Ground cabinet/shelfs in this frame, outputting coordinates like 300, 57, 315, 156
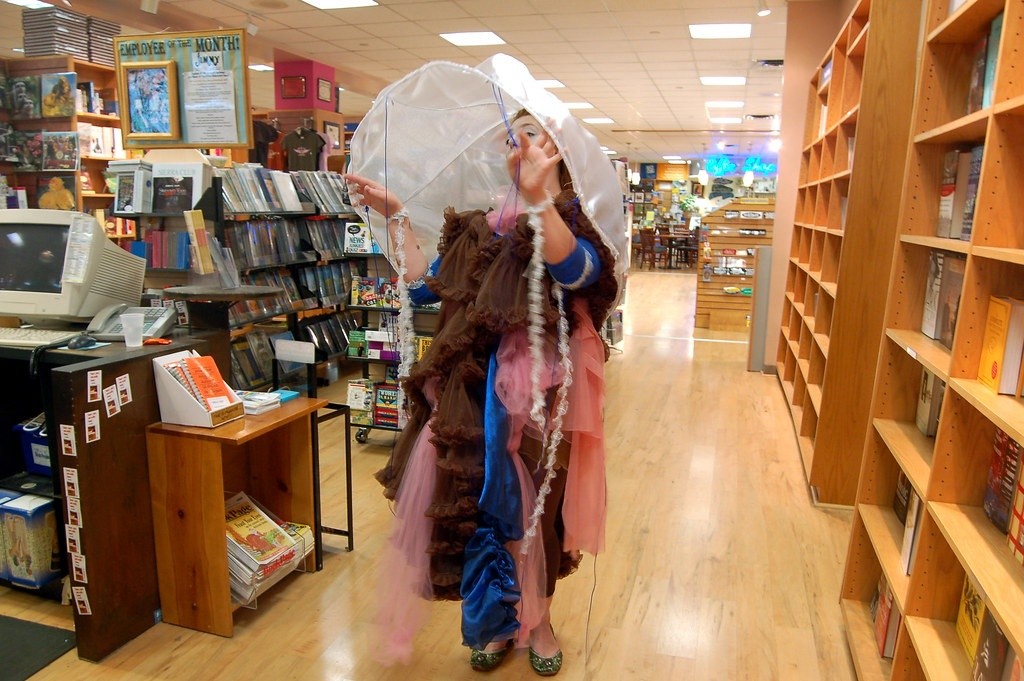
776, 0, 1024, 681
0, 55, 405, 443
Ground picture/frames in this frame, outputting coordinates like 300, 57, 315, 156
318, 77, 332, 102
323, 120, 341, 150
121, 59, 181, 141
281, 76, 305, 99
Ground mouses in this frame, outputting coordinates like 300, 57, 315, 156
68, 335, 97, 349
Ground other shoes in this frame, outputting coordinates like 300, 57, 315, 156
471, 636, 515, 671
529, 622, 563, 676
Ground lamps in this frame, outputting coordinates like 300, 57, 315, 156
244, 14, 258, 36
625, 142, 633, 181
698, 142, 709, 186
756, 0, 771, 17
742, 143, 754, 187
138, 0, 159, 15
632, 147, 640, 185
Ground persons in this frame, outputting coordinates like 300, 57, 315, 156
342, 107, 618, 676
3, 513, 33, 574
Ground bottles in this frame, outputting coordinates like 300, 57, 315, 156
643, 220, 647, 228
640, 218, 643, 229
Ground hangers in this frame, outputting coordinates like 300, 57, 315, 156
271, 119, 281, 134
294, 118, 314, 137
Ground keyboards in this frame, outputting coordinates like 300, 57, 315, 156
0, 327, 82, 347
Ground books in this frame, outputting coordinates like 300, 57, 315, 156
964, 10, 1004, 115
231, 388, 282, 415
956, 573, 1024, 681
981, 425, 1024, 566
867, 568, 902, 660
916, 365, 948, 440
162, 356, 235, 416
920, 250, 968, 351
892, 467, 923, 578
224, 489, 317, 601
936, 142, 985, 242
976, 292, 1024, 398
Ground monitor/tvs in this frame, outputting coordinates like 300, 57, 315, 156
0, 209, 147, 332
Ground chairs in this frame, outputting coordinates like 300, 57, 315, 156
639, 225, 698, 271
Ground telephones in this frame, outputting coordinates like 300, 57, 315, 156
86, 303, 178, 343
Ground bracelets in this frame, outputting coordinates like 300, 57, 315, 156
519, 186, 555, 439
387, 207, 417, 433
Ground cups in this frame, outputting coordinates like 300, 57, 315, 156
119, 313, 144, 347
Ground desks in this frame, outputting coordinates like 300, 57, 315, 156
664, 231, 691, 264
146, 390, 329, 636
650, 234, 694, 270
